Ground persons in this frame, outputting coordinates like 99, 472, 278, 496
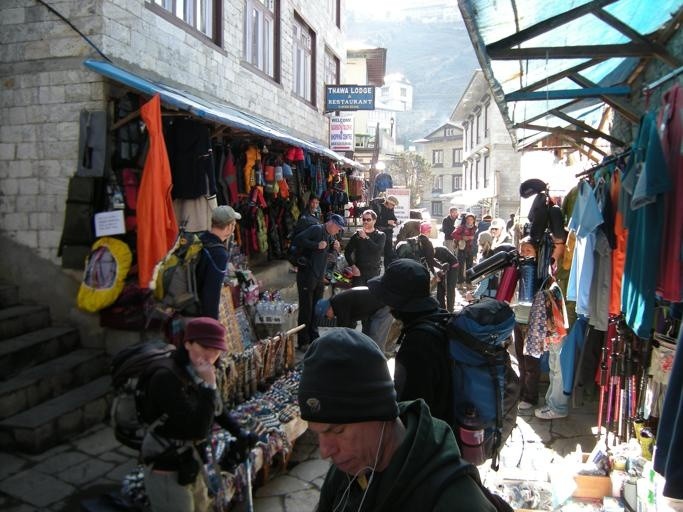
135, 317, 260, 511
293, 325, 515, 512
286, 196, 585, 474
162, 204, 242, 348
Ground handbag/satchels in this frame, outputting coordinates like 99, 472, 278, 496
100, 284, 165, 331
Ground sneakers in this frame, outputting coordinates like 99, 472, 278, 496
535, 406, 567, 419
519, 400, 532, 410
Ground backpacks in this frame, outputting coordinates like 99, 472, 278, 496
103, 338, 177, 449
144, 232, 223, 321
395, 237, 419, 258
453, 212, 477, 249
428, 299, 522, 471
287, 215, 326, 266
369, 198, 385, 210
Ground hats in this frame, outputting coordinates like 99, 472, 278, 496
332, 214, 346, 232
386, 196, 398, 205
212, 205, 242, 225
182, 316, 228, 351
490, 218, 502, 229
314, 299, 331, 317
520, 179, 566, 244
366, 258, 439, 312
420, 223, 431, 233
300, 328, 400, 423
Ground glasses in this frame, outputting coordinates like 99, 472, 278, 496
363, 218, 371, 222
